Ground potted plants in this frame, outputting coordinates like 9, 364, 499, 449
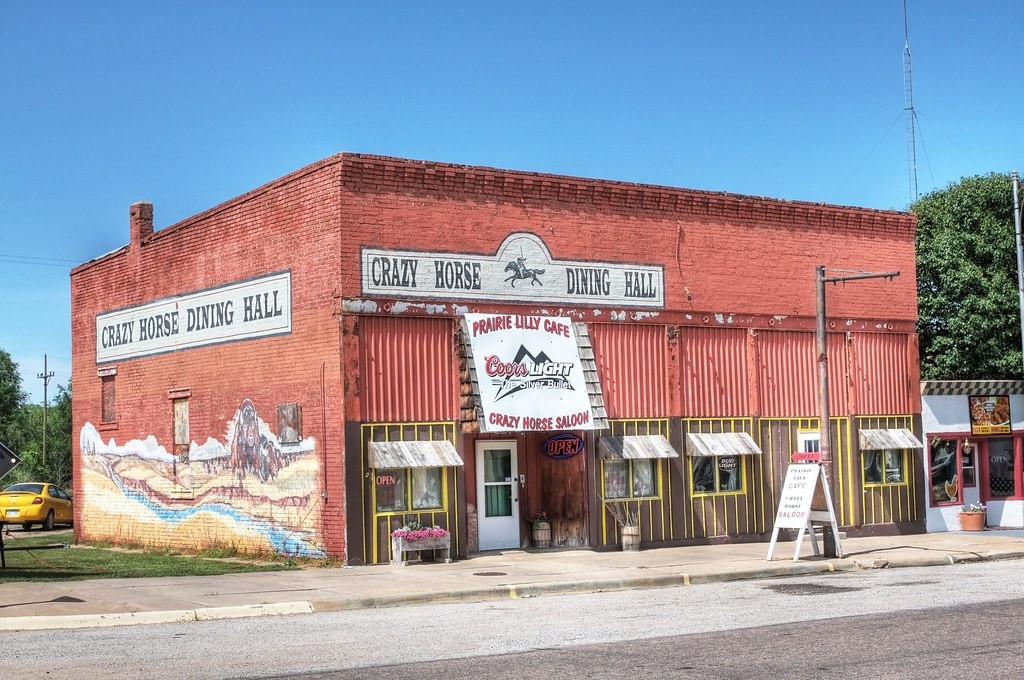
596, 489, 646, 553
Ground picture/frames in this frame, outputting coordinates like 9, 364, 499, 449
968, 394, 1013, 436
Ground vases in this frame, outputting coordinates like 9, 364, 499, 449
390, 533, 454, 567
960, 512, 986, 531
532, 522, 551, 548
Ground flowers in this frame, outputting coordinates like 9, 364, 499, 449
391, 522, 450, 542
526, 512, 551, 528
962, 501, 986, 512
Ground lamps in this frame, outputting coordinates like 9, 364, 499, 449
963, 437, 971, 455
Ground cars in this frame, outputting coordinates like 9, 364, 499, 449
0, 482, 73, 531
